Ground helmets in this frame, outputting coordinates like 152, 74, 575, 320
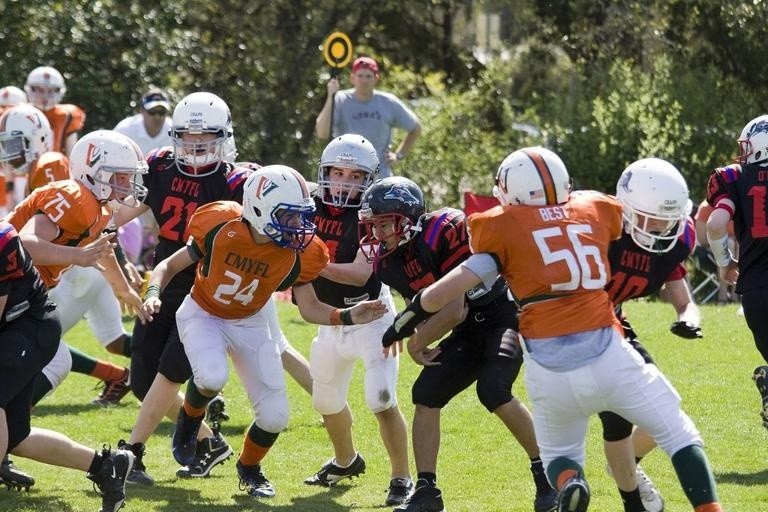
615, 158, 693, 254
355, 176, 425, 263
0, 65, 68, 177
69, 127, 149, 208
318, 132, 380, 210
736, 114, 768, 167
167, 92, 232, 178
493, 147, 569, 207
239, 162, 318, 251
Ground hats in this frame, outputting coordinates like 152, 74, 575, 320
352, 56, 379, 73
141, 89, 170, 111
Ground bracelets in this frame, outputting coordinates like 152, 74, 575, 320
142, 285, 162, 302
704, 230, 732, 267
394, 152, 405, 161
328, 306, 354, 327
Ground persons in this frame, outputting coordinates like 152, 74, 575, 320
312, 56, 424, 184
380, 144, 727, 512
0, 67, 265, 511
137, 164, 389, 499
290, 131, 417, 508
702, 113, 767, 438
597, 156, 706, 512
693, 198, 740, 307
355, 174, 561, 512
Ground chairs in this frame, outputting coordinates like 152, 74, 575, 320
685, 248, 733, 305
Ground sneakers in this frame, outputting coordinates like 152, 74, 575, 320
236, 458, 276, 497
532, 480, 591, 511
92, 365, 233, 512
750, 365, 767, 430
393, 486, 446, 512
306, 452, 365, 485
388, 475, 416, 504
608, 462, 665, 512
1, 458, 35, 492
